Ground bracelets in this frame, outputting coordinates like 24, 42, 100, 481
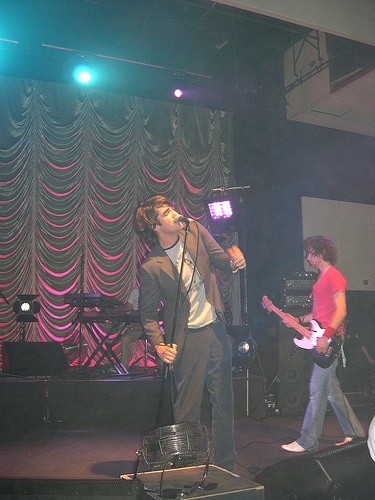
297, 317, 301, 324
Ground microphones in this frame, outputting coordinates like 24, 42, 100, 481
178, 215, 196, 223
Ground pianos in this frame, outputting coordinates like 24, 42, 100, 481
63, 293, 141, 376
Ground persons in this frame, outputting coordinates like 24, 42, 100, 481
109, 287, 167, 374
133, 196, 247, 472
281, 235, 367, 453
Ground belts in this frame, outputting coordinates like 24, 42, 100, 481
189, 319, 221, 332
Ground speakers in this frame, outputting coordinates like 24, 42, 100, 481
1, 339, 68, 377
276, 307, 312, 417
0, 476, 144, 500
252, 436, 375, 500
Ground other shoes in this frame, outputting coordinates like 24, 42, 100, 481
280, 440, 306, 452
334, 436, 354, 446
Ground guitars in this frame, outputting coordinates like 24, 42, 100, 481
260, 295, 342, 369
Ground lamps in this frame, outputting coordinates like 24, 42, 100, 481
13, 294, 41, 323
203, 194, 239, 235
232, 336, 257, 359
134, 422, 212, 496
